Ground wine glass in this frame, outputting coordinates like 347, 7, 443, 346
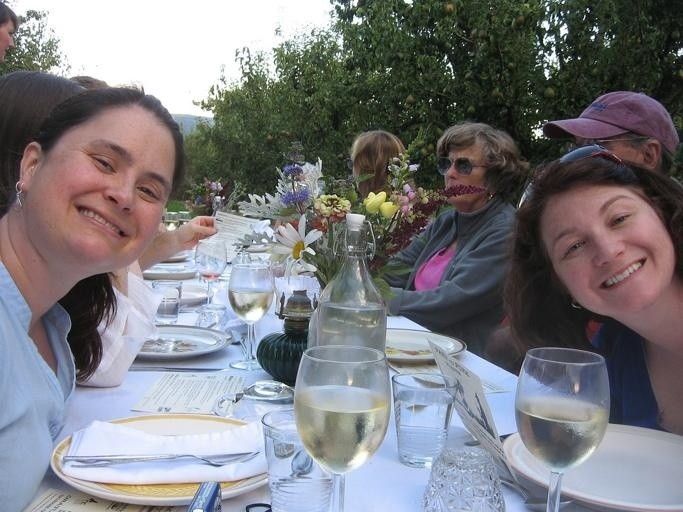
517, 348, 610, 512
296, 345, 392, 512
196, 241, 227, 304
229, 262, 275, 372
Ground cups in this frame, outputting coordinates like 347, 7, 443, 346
154, 279, 181, 326
199, 304, 229, 337
392, 372, 459, 464
426, 447, 506, 512
260, 409, 343, 510
214, 378, 296, 430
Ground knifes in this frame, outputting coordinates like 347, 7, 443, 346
130, 365, 224, 373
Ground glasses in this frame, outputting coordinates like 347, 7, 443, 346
434, 154, 491, 176
515, 143, 638, 211
564, 135, 659, 156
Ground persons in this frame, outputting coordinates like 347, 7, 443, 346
352, 130, 406, 196
69, 76, 219, 271
1, 69, 164, 388
0, 3, 19, 64
514, 146, 681, 431
0, 87, 185, 510
374, 119, 524, 355
486, 90, 681, 381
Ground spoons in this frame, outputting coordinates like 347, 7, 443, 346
61, 451, 255, 467
230, 328, 254, 361
498, 475, 577, 511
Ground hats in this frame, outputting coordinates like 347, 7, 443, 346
539, 87, 683, 157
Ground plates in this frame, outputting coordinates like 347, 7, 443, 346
385, 325, 465, 364
144, 251, 213, 305
137, 323, 230, 363
505, 422, 682, 510
48, 409, 271, 506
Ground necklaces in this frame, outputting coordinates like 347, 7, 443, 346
640, 340, 679, 428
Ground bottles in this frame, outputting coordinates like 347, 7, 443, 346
211, 196, 222, 216
305, 212, 385, 350
